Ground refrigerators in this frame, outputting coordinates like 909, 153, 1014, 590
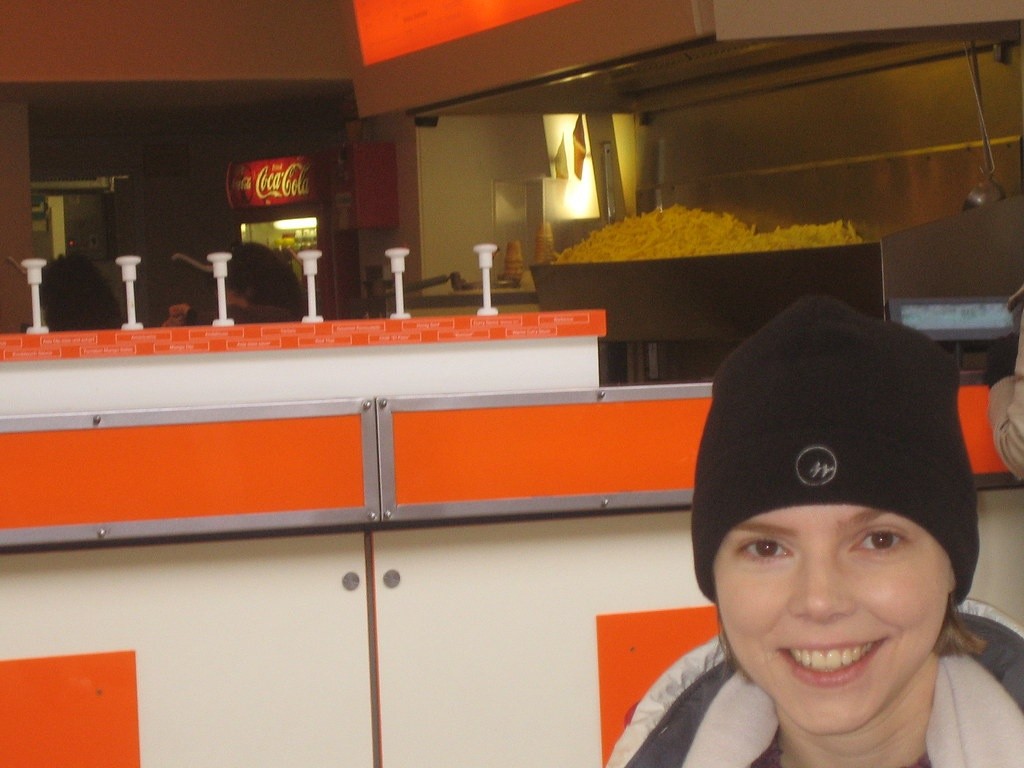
223, 153, 362, 320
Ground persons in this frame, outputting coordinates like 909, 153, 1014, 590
40, 242, 294, 333
605, 295, 1024, 768
985, 284, 1024, 481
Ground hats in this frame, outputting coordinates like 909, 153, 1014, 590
692, 299, 979, 605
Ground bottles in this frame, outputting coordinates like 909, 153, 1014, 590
270, 229, 317, 278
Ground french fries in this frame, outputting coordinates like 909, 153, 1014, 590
552, 204, 862, 263
